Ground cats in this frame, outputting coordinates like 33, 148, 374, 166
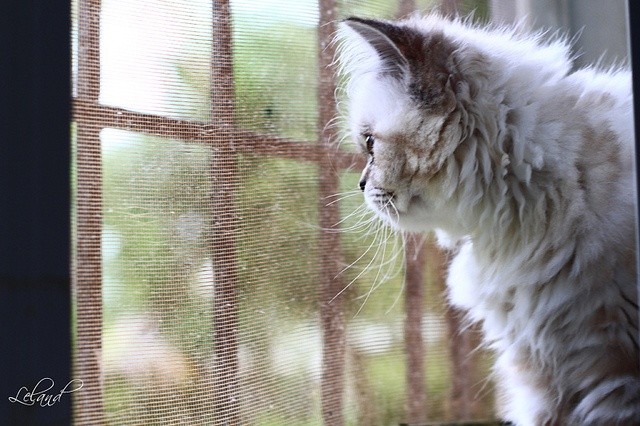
322, 4, 640, 426
